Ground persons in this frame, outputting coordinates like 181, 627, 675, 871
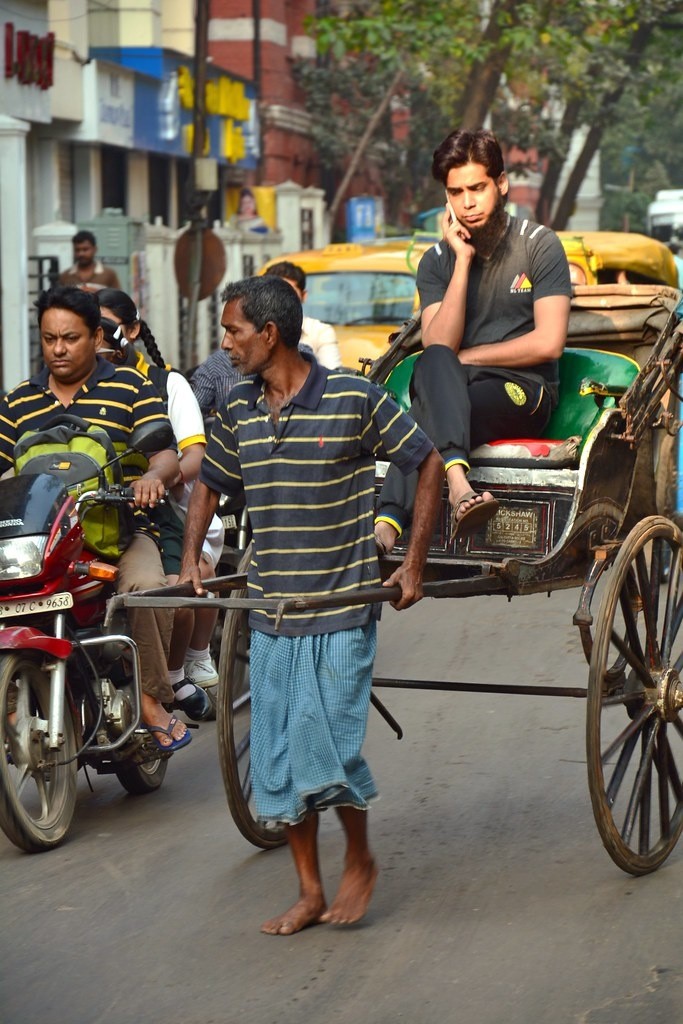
191, 341, 314, 414
58, 229, 122, 296
234, 189, 271, 234
173, 277, 445, 938
263, 262, 342, 371
0, 286, 225, 755
372, 126, 577, 557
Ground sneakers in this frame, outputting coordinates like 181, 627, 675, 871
186, 657, 220, 687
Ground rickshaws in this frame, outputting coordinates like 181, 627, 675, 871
105, 284, 682, 876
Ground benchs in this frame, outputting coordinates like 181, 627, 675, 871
381, 348, 642, 470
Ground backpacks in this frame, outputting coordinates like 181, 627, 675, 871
12, 414, 136, 557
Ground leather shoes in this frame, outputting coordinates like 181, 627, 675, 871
172, 676, 210, 719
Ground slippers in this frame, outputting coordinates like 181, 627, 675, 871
373, 533, 387, 558
140, 715, 193, 752
450, 491, 499, 539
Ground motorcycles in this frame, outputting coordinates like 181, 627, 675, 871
0, 422, 176, 852
253, 190, 683, 370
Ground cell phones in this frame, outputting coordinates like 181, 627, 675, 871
444, 190, 456, 223
